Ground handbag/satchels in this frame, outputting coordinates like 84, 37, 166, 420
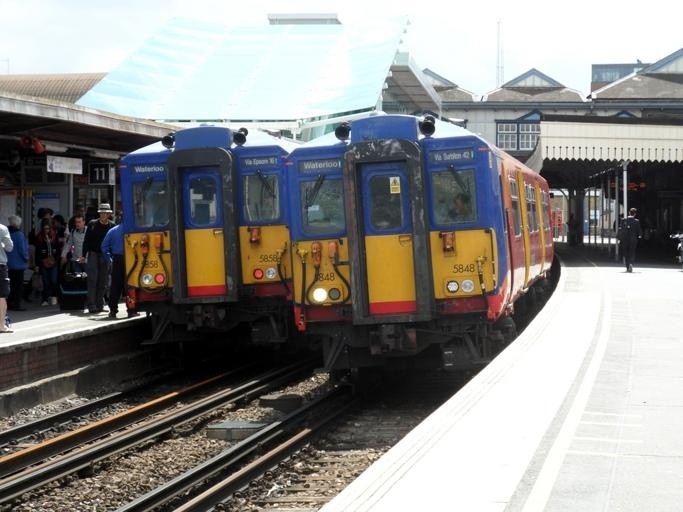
617, 220, 630, 240
43, 258, 53, 268
31, 274, 43, 287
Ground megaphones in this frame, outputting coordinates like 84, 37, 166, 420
32, 138, 45, 154
21, 135, 32, 148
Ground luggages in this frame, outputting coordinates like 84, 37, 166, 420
57, 260, 87, 311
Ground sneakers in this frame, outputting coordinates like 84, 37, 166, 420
51, 296, 57, 305
0, 327, 13, 333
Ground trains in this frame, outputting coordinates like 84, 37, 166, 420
285, 114, 555, 382
120, 125, 305, 368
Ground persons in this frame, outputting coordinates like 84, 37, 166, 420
5, 214, 30, 313
618, 207, 644, 273
447, 193, 476, 222
191, 185, 216, 225
31, 202, 142, 319
0, 223, 15, 334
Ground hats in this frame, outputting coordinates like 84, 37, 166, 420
97, 204, 112, 213
